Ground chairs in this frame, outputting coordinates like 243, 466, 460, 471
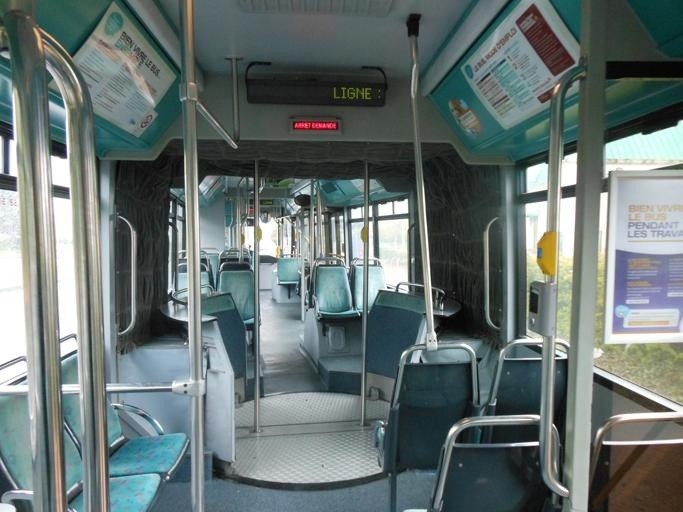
361, 283, 570, 512
588, 412, 683, 511
430, 415, 560, 512
1, 334, 192, 511
277, 253, 387, 334
173, 246, 255, 331
